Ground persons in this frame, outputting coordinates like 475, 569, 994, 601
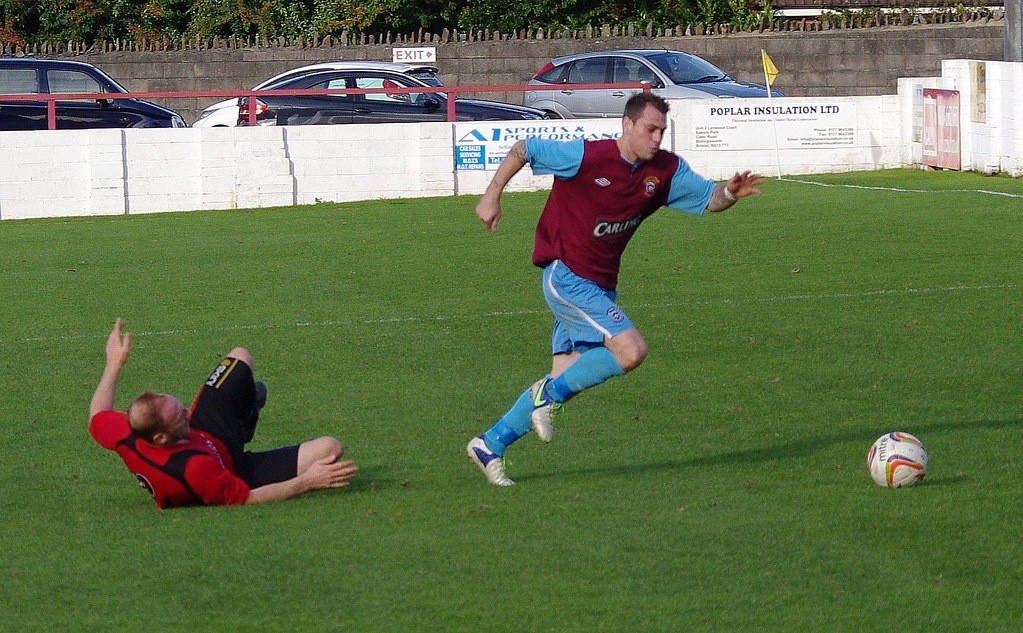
467, 93, 768, 484
87, 318, 357, 507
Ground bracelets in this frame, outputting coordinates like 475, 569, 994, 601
721, 186, 735, 201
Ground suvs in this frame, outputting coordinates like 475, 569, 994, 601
191, 60, 447, 129
233, 67, 551, 128
0, 54, 189, 131
522, 48, 786, 120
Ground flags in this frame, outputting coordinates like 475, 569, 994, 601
762, 50, 778, 86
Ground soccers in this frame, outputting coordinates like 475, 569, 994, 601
867, 431, 928, 488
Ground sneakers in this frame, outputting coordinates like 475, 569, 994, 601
466, 435, 514, 488
530, 375, 564, 443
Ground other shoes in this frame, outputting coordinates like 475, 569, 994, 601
254, 381, 267, 408
